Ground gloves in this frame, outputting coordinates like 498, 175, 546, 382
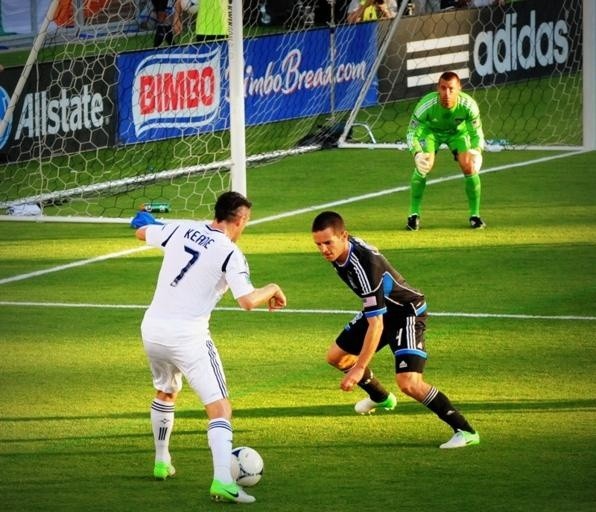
468, 149, 482, 172
415, 153, 430, 172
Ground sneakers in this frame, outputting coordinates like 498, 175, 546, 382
439, 431, 480, 449
408, 214, 419, 231
209, 480, 256, 504
469, 216, 485, 229
354, 392, 397, 415
154, 462, 175, 480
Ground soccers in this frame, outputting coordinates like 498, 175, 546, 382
227, 446, 263, 485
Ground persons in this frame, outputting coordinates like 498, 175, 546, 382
135, 192, 287, 503
311, 211, 480, 449
407, 71, 486, 230
244, 1, 398, 32
52, 0, 233, 47
